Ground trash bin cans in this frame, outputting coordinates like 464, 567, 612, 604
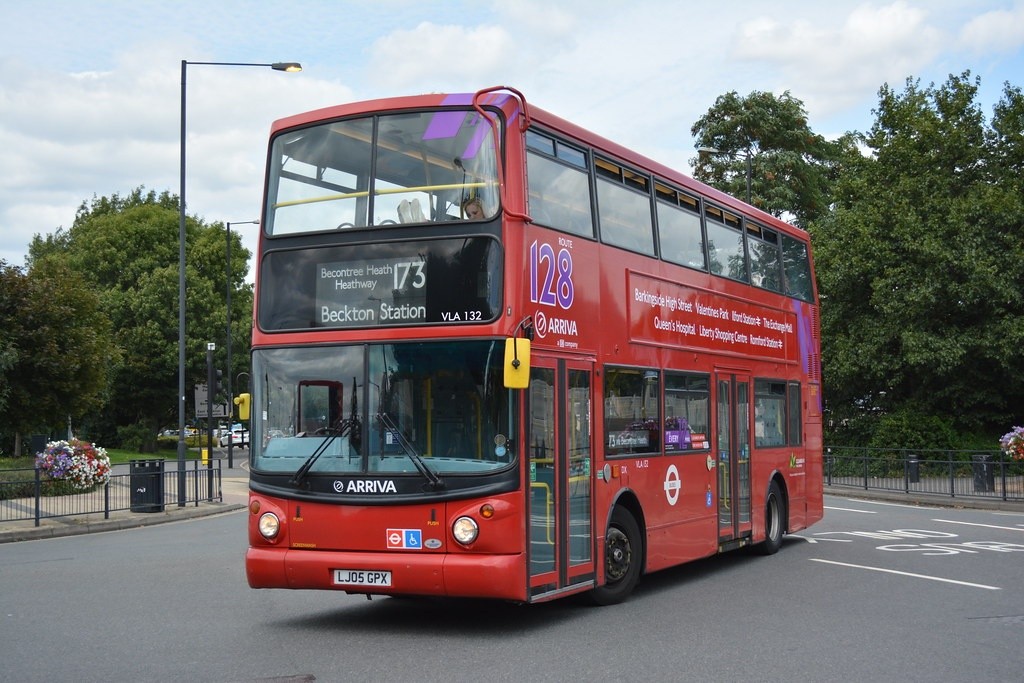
909, 454, 919, 484
129, 459, 165, 513
972, 454, 995, 492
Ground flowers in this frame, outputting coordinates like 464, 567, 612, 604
998, 427, 1024, 460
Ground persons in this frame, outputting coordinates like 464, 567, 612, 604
464, 197, 494, 221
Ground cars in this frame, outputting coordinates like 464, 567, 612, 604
158, 419, 251, 449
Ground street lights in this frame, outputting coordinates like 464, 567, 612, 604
177, 58, 302, 508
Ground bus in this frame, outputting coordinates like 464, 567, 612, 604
232, 84, 824, 607
